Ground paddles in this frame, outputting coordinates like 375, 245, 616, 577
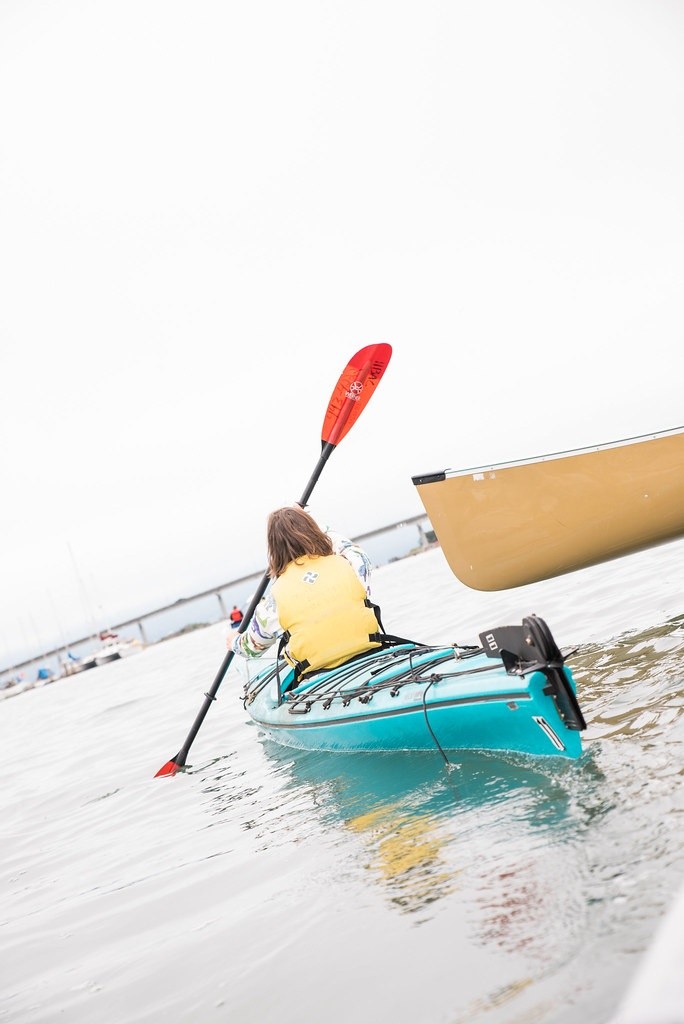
152, 342, 397, 780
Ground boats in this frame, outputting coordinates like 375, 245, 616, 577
237, 617, 589, 764
410, 423, 682, 593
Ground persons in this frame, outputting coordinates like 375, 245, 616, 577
230, 605, 243, 623
226, 506, 385, 693
